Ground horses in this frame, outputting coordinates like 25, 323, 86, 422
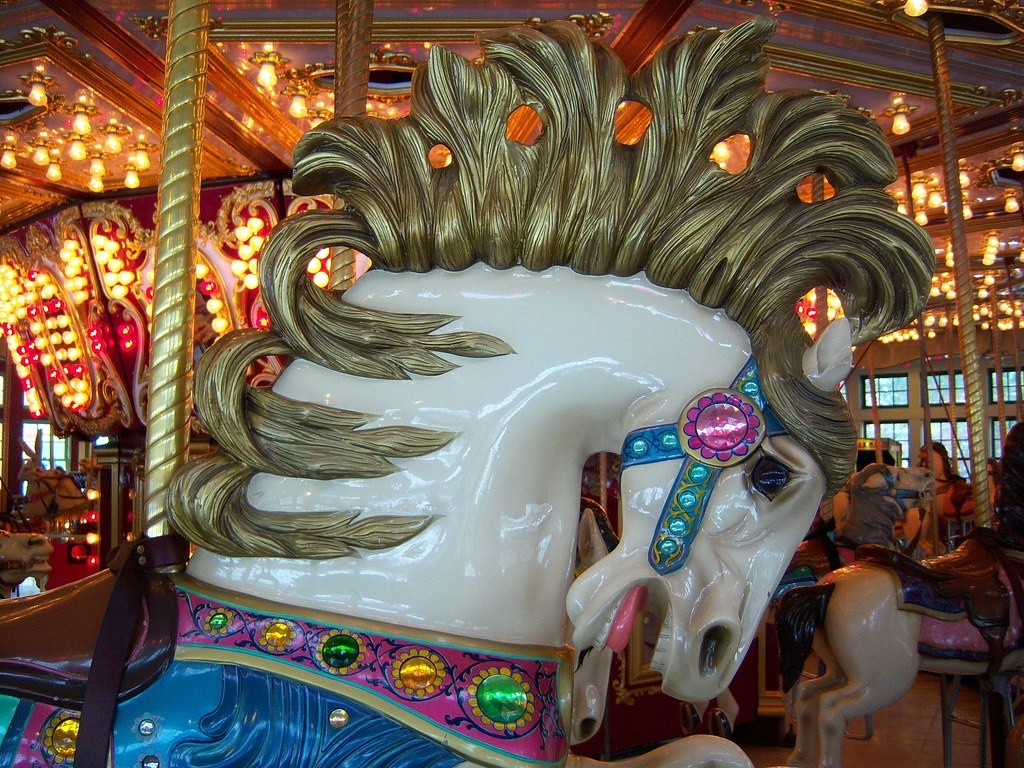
0, 15, 1024, 768
